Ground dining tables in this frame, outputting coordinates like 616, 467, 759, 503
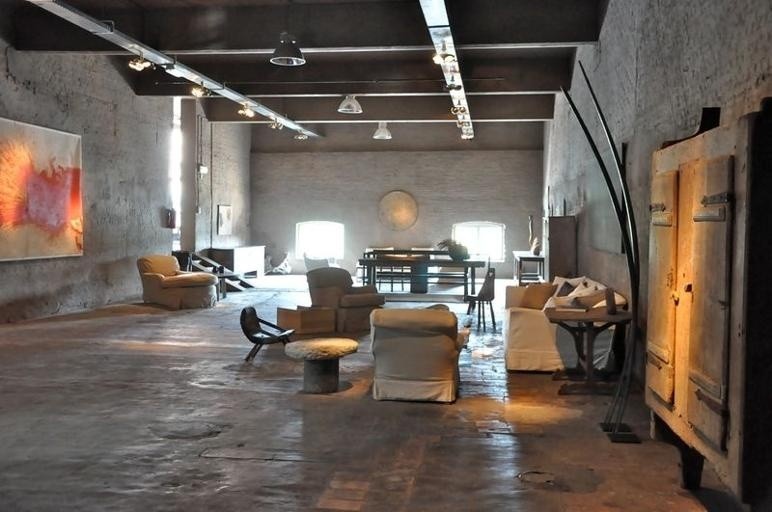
438, 239, 467, 261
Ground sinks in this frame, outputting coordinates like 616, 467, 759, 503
371, 308, 467, 405
306, 267, 384, 338
502, 277, 626, 373
136, 253, 219, 310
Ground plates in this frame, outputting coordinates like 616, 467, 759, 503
544, 216, 577, 282
644, 95, 772, 511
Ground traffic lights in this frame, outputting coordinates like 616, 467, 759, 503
543, 282, 586, 310
574, 289, 606, 307
520, 282, 557, 309
556, 281, 574, 297
553, 285, 596, 309
551, 276, 586, 296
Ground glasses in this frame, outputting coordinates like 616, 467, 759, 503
237, 102, 255, 118
442, 76, 462, 93
129, 54, 151, 71
269, 31, 306, 66
338, 97, 363, 114
432, 44, 455, 65
192, 82, 204, 97
267, 120, 283, 130
451, 100, 466, 115
373, 123, 392, 140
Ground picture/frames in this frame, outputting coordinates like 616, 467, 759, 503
545, 308, 631, 394
208, 246, 266, 278
513, 249, 544, 285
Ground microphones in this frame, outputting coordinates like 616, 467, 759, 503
285, 336, 357, 393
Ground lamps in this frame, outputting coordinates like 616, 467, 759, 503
464, 267, 495, 328
240, 307, 296, 361
